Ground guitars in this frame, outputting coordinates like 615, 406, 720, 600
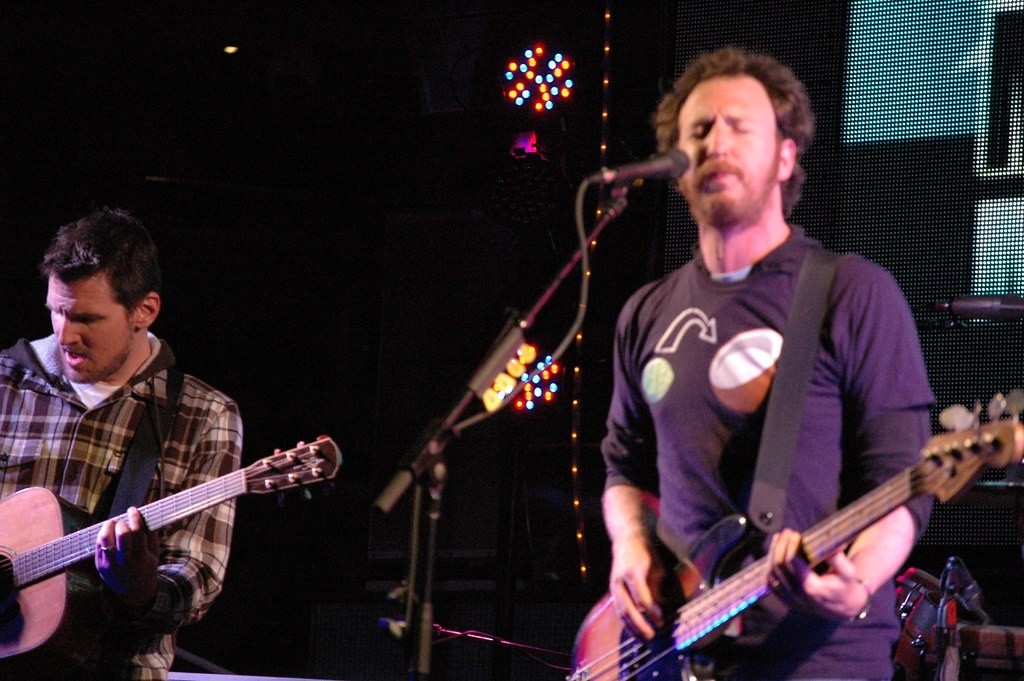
1, 434, 341, 662
566, 389, 1024, 681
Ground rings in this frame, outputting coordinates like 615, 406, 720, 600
98, 543, 116, 554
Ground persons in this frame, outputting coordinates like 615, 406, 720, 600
0, 212, 243, 681
601, 48, 933, 681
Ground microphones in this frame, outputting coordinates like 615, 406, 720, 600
589, 149, 689, 183
950, 558, 985, 611
937, 295, 1024, 322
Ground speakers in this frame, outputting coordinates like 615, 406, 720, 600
312, 208, 611, 681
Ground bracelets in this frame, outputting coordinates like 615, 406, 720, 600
846, 580, 874, 627
